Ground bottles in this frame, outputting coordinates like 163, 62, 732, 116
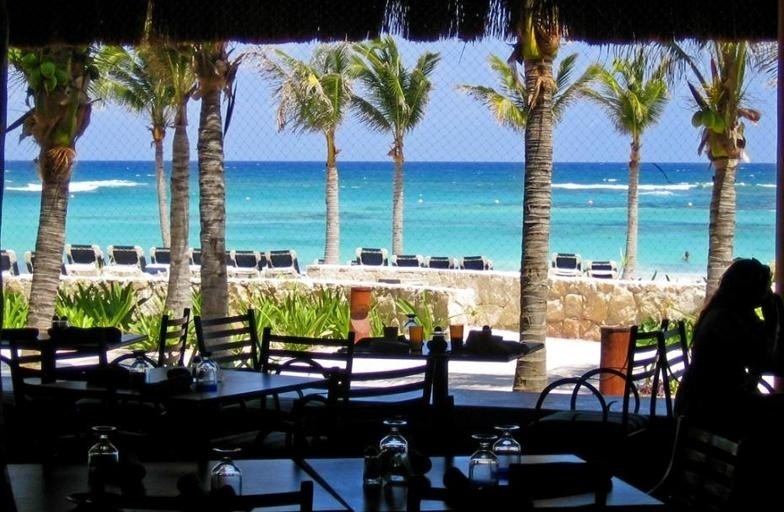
46, 316, 68, 336
128, 347, 222, 395
427, 326, 447, 351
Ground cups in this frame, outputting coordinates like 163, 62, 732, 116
448, 324, 463, 347
382, 326, 398, 341
408, 325, 424, 352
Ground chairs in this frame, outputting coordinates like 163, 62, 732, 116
0, 316, 436, 458
0, 243, 617, 281
530, 316, 783, 511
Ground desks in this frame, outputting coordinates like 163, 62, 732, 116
6, 458, 350, 511
303, 454, 665, 511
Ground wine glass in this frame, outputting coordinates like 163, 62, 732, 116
208, 447, 244, 496
85, 424, 120, 488
375, 417, 407, 463
490, 424, 524, 466
467, 431, 500, 482
403, 313, 418, 340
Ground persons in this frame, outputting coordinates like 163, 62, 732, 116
675, 259, 780, 499
